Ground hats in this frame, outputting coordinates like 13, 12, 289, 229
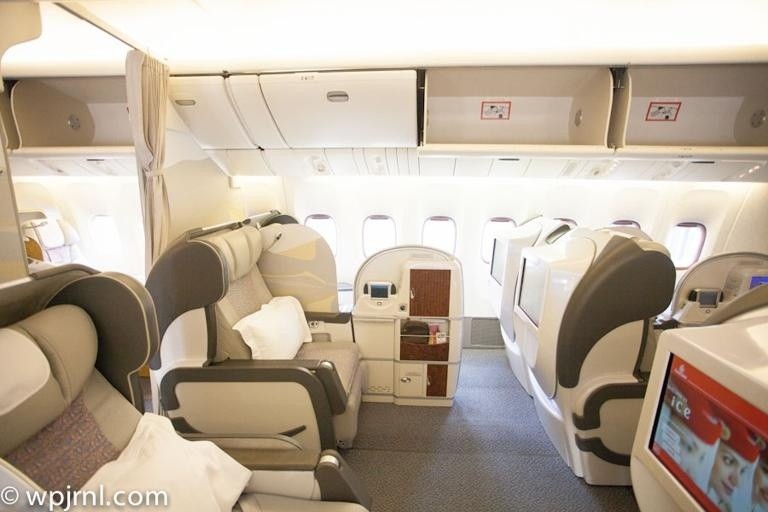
665, 382, 760, 462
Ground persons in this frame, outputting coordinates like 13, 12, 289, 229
668, 409, 768, 512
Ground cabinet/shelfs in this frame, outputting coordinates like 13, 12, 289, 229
349, 246, 465, 407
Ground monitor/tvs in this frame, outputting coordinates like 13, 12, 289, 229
514, 249, 551, 336
634, 331, 768, 512
488, 236, 509, 289
